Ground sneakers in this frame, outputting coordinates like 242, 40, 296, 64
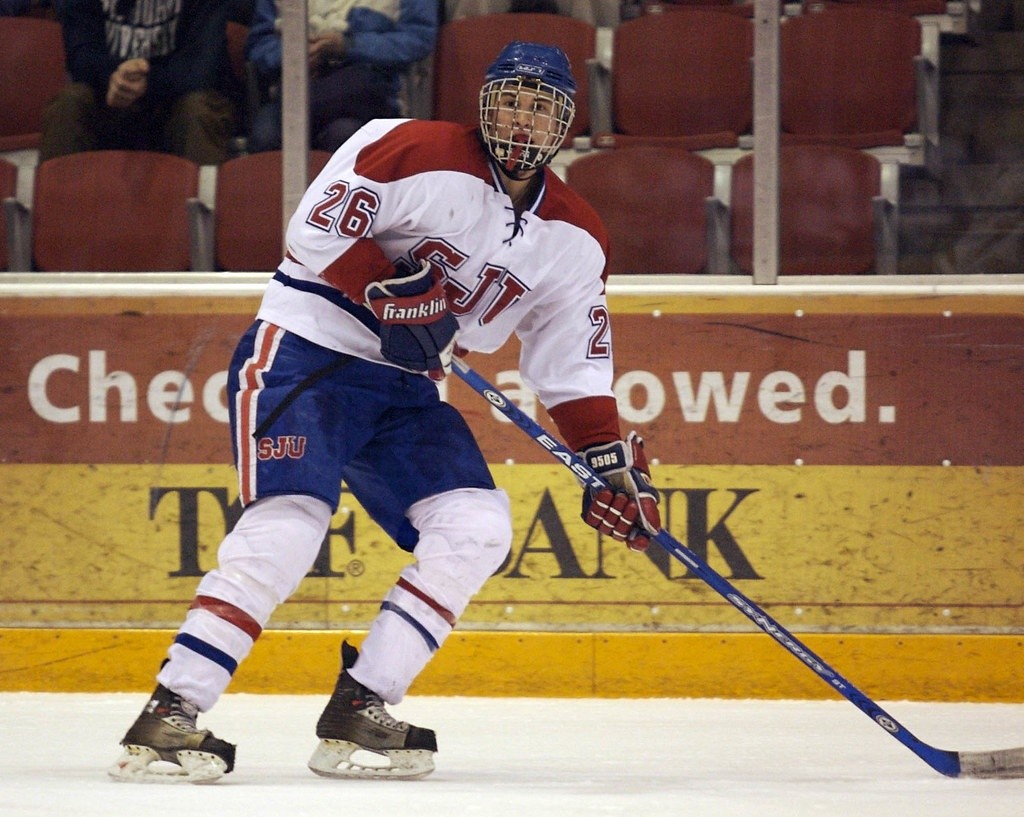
307, 639, 439, 781
106, 657, 237, 784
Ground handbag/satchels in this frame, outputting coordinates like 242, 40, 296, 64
307, 52, 402, 120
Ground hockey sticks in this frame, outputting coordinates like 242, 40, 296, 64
448, 352, 1024, 784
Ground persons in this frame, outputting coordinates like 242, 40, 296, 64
109, 39, 663, 773
41, 1, 441, 168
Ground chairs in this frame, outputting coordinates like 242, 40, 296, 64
32, 150, 217, 273
595, 13, 755, 151
728, 143, 898, 275
0, 16, 80, 153
0, 159, 34, 272
565, 145, 730, 273
780, 11, 943, 179
215, 150, 330, 272
433, 15, 612, 149
802, 0, 984, 43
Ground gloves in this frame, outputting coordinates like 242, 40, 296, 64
574, 430, 661, 553
362, 258, 461, 383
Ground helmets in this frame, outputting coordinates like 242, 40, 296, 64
478, 40, 577, 181
